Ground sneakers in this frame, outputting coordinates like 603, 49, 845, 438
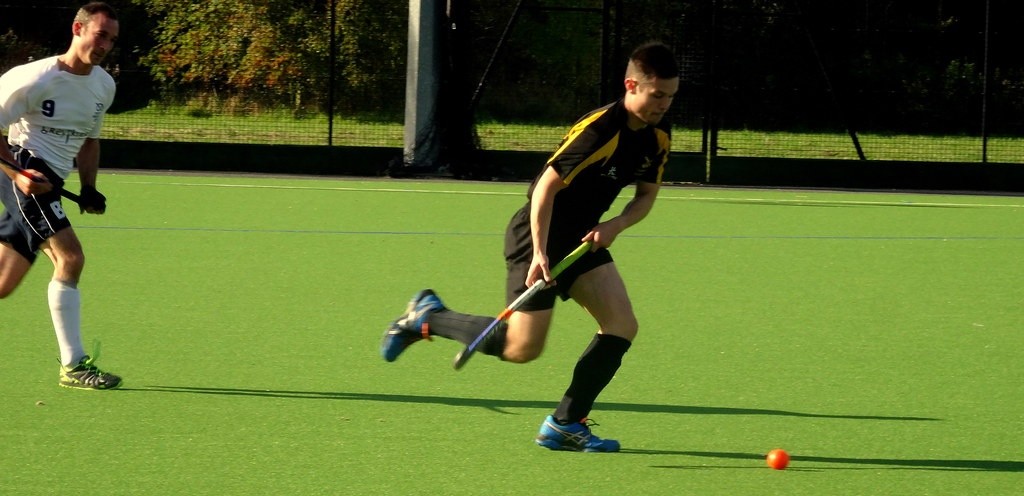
56, 339, 124, 392
380, 289, 445, 362
534, 415, 620, 454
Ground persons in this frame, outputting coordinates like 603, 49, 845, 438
0, 3, 123, 390
384, 43, 681, 453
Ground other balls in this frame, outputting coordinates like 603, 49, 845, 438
766, 448, 789, 470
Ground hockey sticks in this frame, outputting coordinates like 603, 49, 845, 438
451, 238, 594, 371
0, 156, 85, 206
